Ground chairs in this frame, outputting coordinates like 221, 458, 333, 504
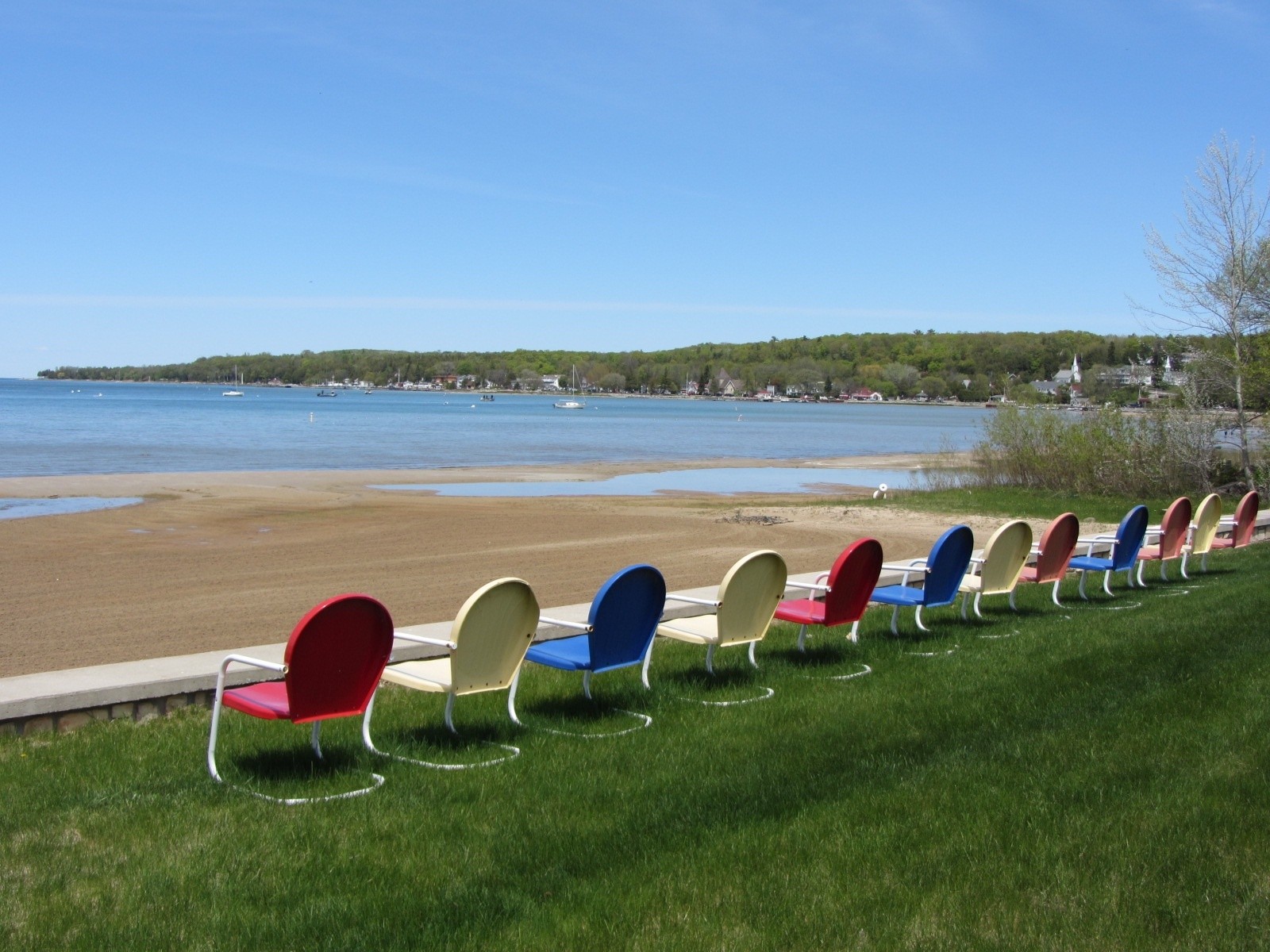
853, 524, 976, 659
1077, 503, 1151, 613
363, 578, 538, 769
1210, 491, 1260, 583
1135, 498, 1191, 602
959, 512, 1085, 627
747, 538, 883, 683
1177, 495, 1222, 589
507, 566, 665, 742
203, 593, 396, 805
643, 549, 788, 708
914, 520, 1033, 639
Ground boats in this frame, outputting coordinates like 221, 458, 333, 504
479, 393, 495, 402
364, 390, 373, 395
317, 390, 337, 397
985, 395, 1008, 408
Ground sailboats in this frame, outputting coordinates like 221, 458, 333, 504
222, 364, 244, 397
552, 363, 587, 409
